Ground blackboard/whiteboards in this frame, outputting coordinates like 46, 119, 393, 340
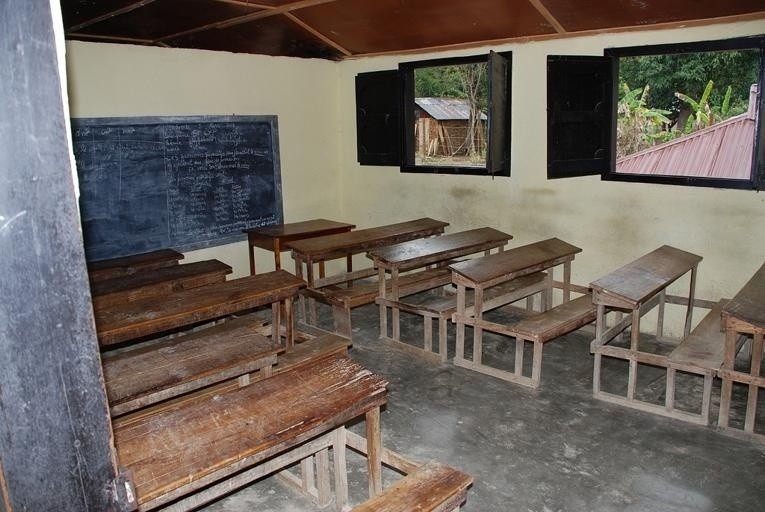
71, 115, 283, 264
